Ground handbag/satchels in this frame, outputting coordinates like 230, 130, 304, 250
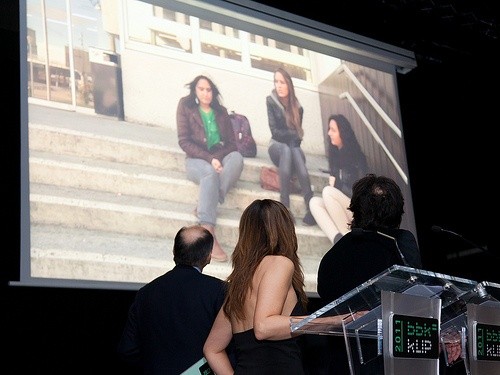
260, 167, 298, 193
229, 111, 256, 158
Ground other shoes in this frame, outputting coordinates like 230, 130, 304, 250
200, 224, 228, 262
302, 211, 316, 226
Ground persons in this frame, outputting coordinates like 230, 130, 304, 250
318, 177, 421, 308
120, 228, 235, 375
175, 75, 244, 263
309, 114, 366, 246
268, 70, 319, 228
203, 200, 372, 375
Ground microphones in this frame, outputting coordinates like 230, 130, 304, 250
352, 228, 409, 267
431, 225, 486, 252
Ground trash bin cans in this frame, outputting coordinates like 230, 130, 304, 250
89, 59, 119, 116
